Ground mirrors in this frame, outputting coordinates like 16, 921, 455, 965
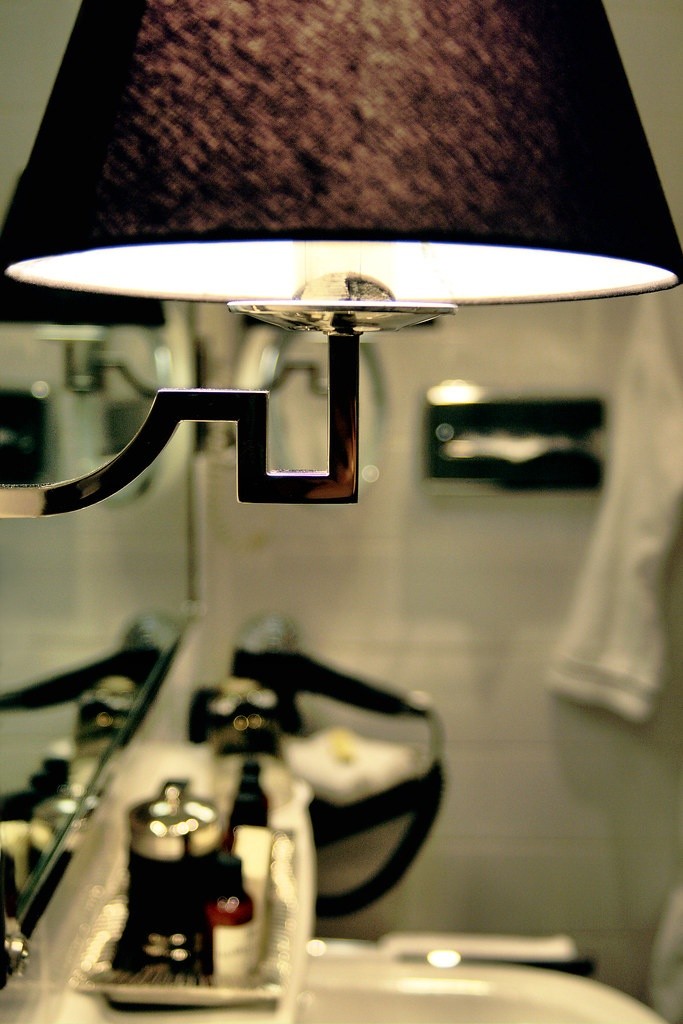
0, 0, 199, 986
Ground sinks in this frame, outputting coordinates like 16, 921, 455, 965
294, 942, 670, 1022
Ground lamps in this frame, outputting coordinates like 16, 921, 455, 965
0, 0, 683, 520
